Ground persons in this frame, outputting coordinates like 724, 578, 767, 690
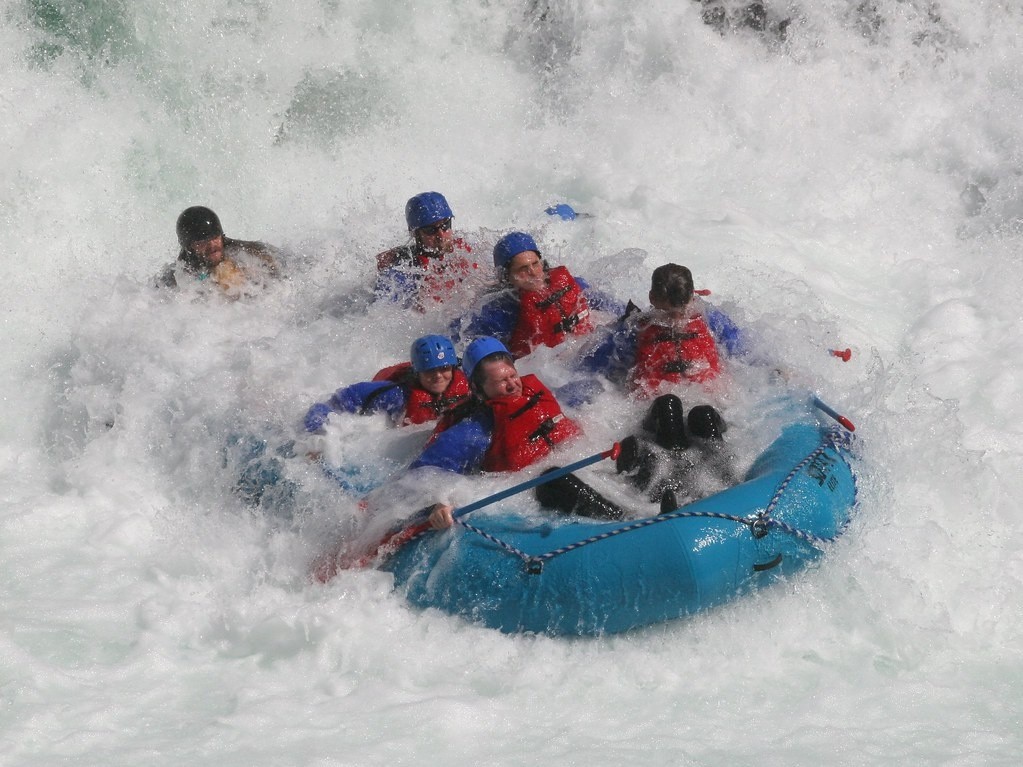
306, 335, 470, 426
377, 190, 470, 315
154, 204, 279, 288
577, 263, 742, 451
450, 232, 619, 359
409, 337, 676, 531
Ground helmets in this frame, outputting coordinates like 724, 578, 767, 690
493, 232, 541, 267
462, 337, 511, 381
176, 206, 223, 241
405, 191, 455, 231
545, 204, 576, 221
410, 334, 459, 373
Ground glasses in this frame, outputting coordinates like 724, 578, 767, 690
418, 219, 450, 236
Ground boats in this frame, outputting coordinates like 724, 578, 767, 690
222, 330, 888, 638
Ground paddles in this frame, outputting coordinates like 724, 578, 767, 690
813, 396, 855, 432
317, 443, 622, 581
829, 348, 851, 362
694, 289, 711, 295
